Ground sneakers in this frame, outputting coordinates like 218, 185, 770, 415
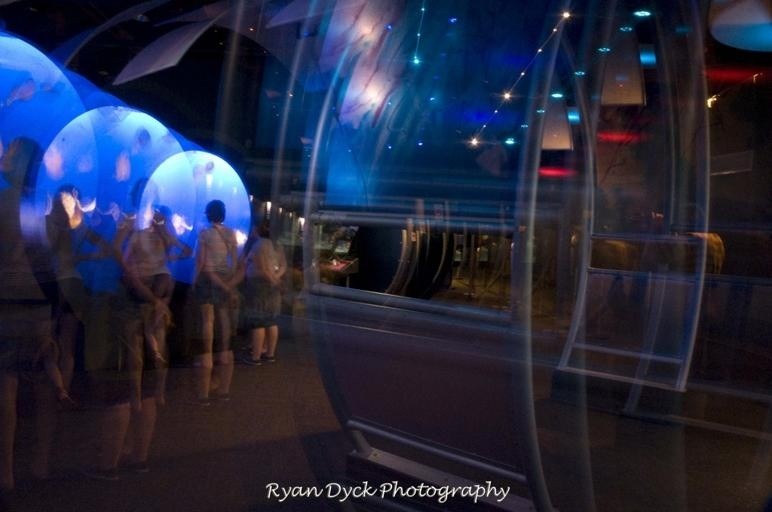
184, 345, 276, 407
82, 459, 150, 482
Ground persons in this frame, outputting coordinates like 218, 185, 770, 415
2, 134, 771, 485
